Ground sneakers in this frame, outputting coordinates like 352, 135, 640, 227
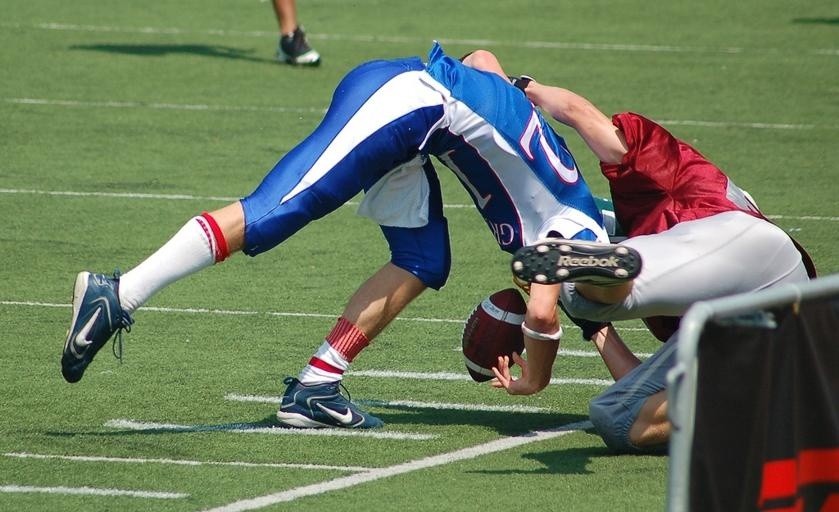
512, 236, 642, 288
277, 26, 320, 65
62, 267, 134, 383
277, 375, 385, 429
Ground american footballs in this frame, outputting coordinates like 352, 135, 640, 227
461, 289, 527, 382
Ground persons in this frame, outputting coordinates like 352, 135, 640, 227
274, 0, 320, 65
61, 38, 612, 432
504, 68, 818, 455
